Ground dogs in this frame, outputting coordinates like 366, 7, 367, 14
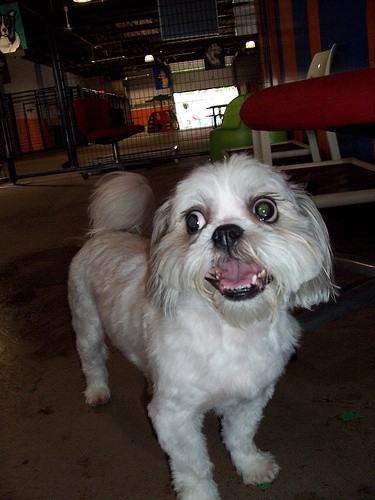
66, 149, 341, 500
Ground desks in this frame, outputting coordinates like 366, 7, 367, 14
207, 104, 229, 129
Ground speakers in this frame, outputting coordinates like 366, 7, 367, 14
157, 0, 219, 42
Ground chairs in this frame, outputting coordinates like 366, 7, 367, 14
239, 0, 375, 208
250, 42, 342, 169
209, 93, 288, 162
73, 97, 159, 179
